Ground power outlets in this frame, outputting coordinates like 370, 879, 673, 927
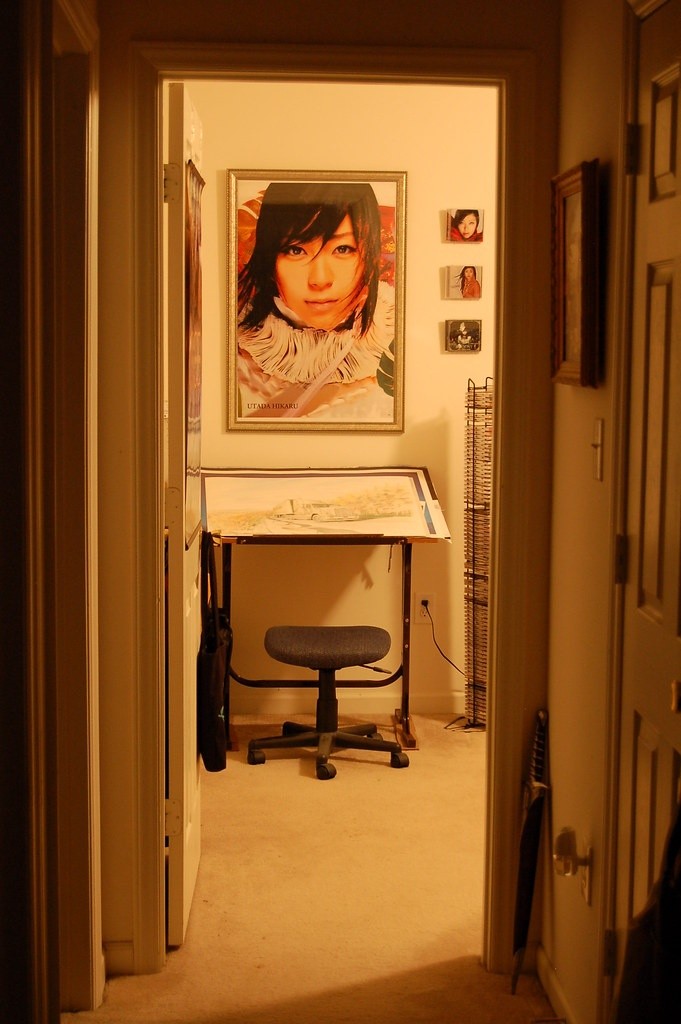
415, 593, 434, 624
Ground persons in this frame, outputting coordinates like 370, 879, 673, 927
453, 266, 480, 299
451, 209, 483, 242
458, 322, 477, 351
238, 184, 395, 422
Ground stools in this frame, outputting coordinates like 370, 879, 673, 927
250, 626, 410, 779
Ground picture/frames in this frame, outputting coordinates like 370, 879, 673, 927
226, 168, 406, 431
548, 159, 607, 390
